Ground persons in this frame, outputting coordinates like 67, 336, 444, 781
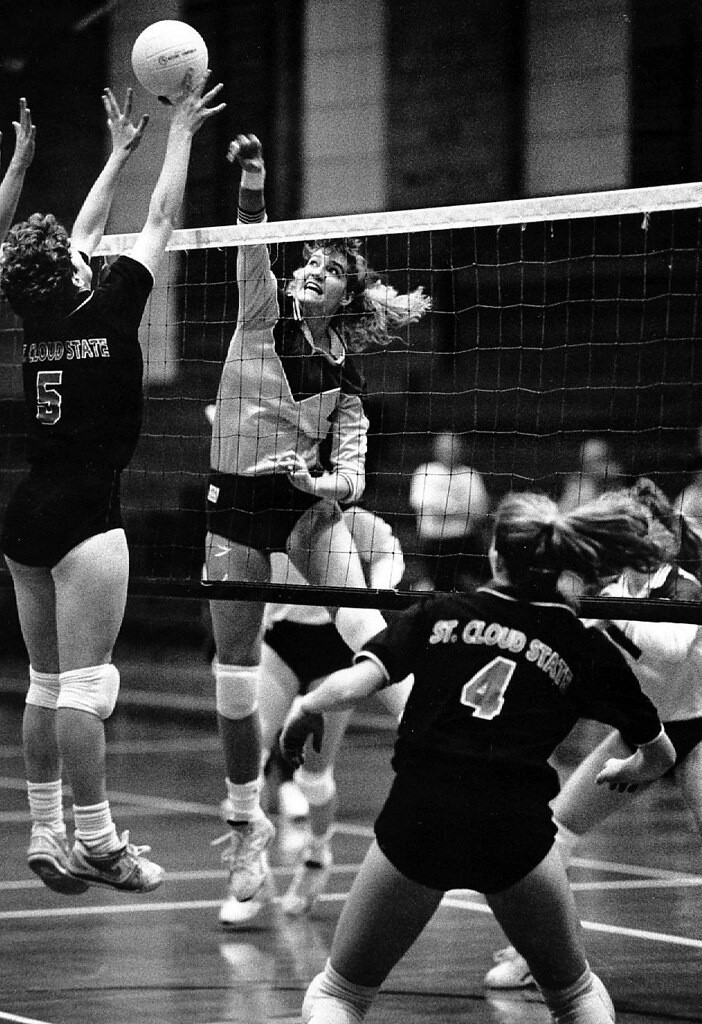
0, 96, 38, 245
277, 492, 675, 1024
0, 69, 226, 895
485, 478, 702, 988
557, 437, 622, 602
260, 503, 404, 917
673, 460, 701, 581
407, 430, 493, 593
201, 130, 418, 901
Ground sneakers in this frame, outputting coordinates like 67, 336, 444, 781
280, 848, 334, 919
26, 831, 90, 896
217, 864, 275, 925
210, 800, 277, 904
482, 945, 534, 989
66, 829, 166, 894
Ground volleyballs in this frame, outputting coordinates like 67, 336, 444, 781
130, 20, 209, 100
